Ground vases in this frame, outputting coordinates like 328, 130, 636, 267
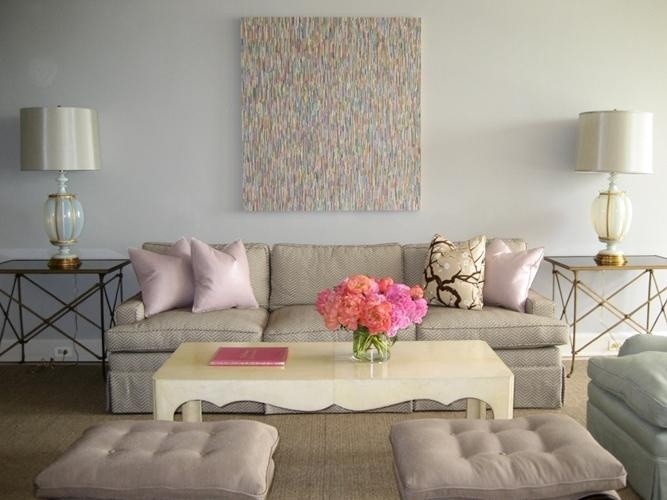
342, 321, 399, 365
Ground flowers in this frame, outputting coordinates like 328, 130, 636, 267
315, 275, 431, 364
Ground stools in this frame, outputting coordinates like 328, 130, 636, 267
586, 333, 666, 499
389, 416, 628, 499
33, 420, 280, 499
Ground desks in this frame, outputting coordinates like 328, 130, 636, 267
544, 255, 667, 378
1, 259, 130, 391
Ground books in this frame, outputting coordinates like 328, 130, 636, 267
208, 347, 288, 366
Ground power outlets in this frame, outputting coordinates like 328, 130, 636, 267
55, 345, 73, 359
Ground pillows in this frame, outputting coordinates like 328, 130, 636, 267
191, 241, 260, 316
486, 237, 512, 257
123, 242, 192, 316
482, 248, 545, 313
421, 236, 488, 311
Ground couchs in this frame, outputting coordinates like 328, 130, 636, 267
100, 239, 565, 411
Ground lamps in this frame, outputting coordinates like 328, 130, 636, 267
18, 105, 104, 269
574, 111, 652, 267
